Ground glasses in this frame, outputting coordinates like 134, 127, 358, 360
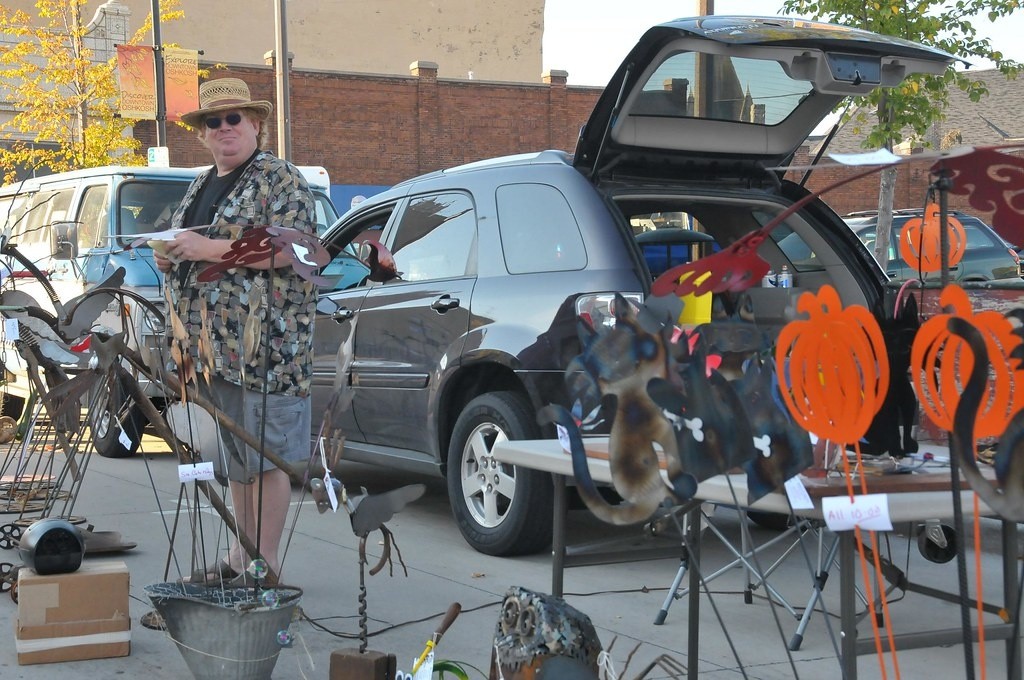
205, 114, 241, 129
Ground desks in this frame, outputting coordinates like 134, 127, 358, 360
494, 436, 1021, 680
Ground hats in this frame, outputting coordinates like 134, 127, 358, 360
181, 78, 273, 132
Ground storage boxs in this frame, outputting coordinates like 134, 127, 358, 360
15, 561, 131, 665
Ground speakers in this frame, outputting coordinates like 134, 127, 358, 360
19, 517, 84, 576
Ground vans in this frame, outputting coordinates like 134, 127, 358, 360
0, 165, 368, 454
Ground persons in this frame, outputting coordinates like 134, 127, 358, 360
146, 77, 319, 589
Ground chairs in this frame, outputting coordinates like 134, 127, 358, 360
99, 208, 137, 248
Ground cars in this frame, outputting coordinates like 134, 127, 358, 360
837, 206, 1022, 343
312, 14, 932, 564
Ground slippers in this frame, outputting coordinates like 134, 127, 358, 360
219, 569, 276, 588
176, 559, 238, 587
